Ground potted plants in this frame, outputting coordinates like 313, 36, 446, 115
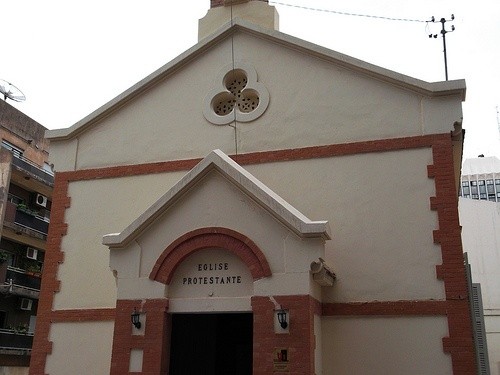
16, 203, 26, 212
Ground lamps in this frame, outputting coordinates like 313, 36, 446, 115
276, 305, 287, 329
130, 307, 141, 329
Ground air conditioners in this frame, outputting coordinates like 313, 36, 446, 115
19, 297, 33, 311
25, 247, 38, 260
34, 193, 48, 208
